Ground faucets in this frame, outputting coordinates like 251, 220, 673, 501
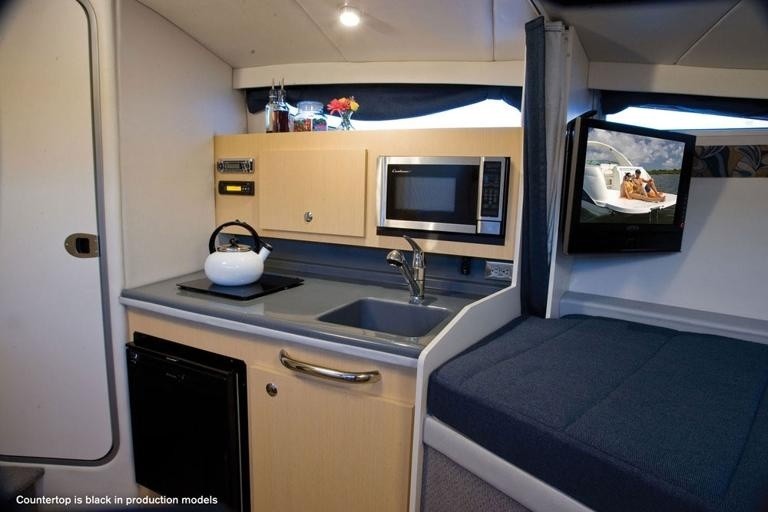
387, 234, 426, 305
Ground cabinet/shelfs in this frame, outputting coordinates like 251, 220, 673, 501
254, 136, 373, 243
241, 334, 416, 512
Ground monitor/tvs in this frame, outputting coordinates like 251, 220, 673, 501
563, 115, 698, 255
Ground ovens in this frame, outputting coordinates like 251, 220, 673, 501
123, 330, 252, 512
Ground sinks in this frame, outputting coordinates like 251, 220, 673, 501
312, 294, 453, 340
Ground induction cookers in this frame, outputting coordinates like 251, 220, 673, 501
174, 272, 305, 300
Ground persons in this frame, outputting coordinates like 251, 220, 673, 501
632, 169, 666, 202
622, 172, 659, 203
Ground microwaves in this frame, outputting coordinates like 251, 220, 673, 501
373, 153, 510, 248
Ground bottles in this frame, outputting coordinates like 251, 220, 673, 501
264, 89, 289, 132
294, 101, 328, 131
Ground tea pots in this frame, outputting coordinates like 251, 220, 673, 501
203, 219, 273, 287
